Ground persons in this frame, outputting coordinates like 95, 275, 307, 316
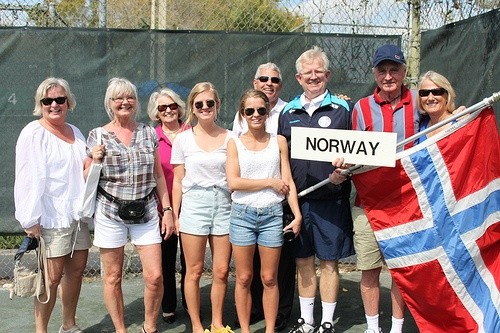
168, 81, 241, 333
225, 89, 303, 333
13, 77, 93, 333
410, 71, 474, 332
83, 76, 175, 332
144, 86, 202, 324
229, 60, 353, 333
278, 48, 356, 333
329, 45, 471, 333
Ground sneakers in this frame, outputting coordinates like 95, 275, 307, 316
287, 318, 317, 333
315, 322, 336, 333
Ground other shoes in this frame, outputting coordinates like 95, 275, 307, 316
163, 313, 175, 324
58, 325, 84, 333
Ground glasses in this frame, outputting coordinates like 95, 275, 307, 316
158, 103, 178, 112
195, 100, 215, 109
245, 107, 267, 116
257, 76, 281, 83
41, 96, 67, 105
419, 88, 445, 97
110, 96, 136, 103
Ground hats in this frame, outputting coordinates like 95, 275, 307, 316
372, 44, 407, 68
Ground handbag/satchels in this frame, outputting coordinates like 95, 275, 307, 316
118, 199, 146, 220
13, 249, 45, 298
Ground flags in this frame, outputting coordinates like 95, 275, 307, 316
354, 105, 499, 333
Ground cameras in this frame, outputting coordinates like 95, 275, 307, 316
282, 213, 296, 243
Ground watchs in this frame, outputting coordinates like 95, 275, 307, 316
162, 206, 173, 212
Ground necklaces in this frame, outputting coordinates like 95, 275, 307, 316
381, 91, 404, 104
161, 122, 181, 134
